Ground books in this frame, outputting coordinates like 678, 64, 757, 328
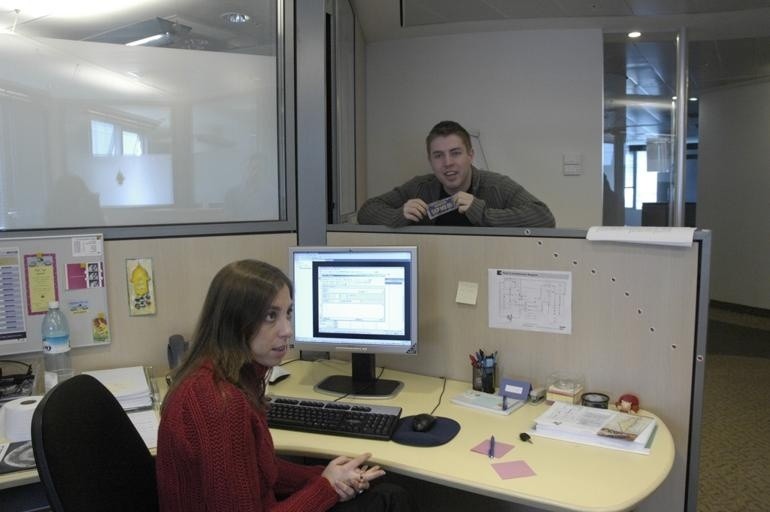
449, 390, 524, 416
526, 402, 660, 453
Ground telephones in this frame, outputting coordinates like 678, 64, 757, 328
167, 334, 190, 381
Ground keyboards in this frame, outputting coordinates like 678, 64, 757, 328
266, 396, 402, 441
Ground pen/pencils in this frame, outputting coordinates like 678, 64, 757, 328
470, 349, 498, 393
489, 434, 494, 458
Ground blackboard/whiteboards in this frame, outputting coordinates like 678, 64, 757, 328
0, 233, 112, 357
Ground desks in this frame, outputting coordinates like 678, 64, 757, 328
0, 359, 675, 512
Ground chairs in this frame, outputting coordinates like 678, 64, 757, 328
31, 374, 155, 512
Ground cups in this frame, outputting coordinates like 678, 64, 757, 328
47, 367, 75, 383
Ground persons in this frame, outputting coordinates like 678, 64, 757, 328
356, 120, 556, 227
155, 259, 386, 511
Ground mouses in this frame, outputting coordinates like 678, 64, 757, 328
412, 414, 435, 432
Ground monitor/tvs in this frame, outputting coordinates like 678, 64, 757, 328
288, 246, 419, 399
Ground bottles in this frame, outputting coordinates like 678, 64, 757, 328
40, 299, 73, 370
